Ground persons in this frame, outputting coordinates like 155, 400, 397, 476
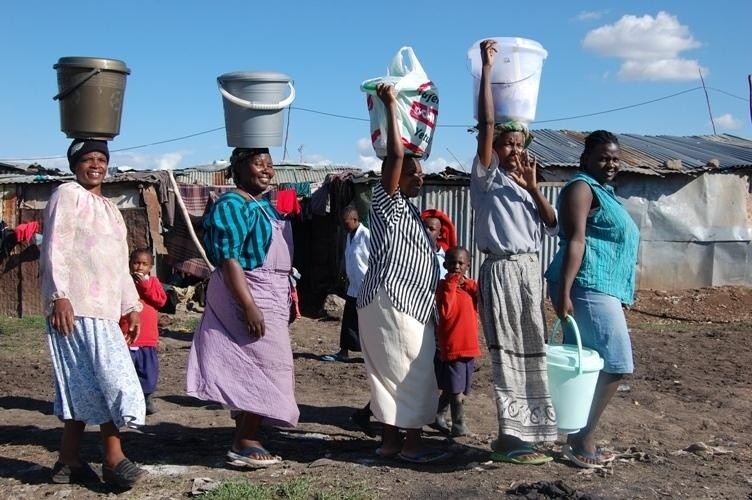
355, 81, 448, 464
547, 131, 640, 469
122, 248, 166, 414
436, 245, 480, 435
322, 206, 373, 362
471, 39, 559, 463
183, 145, 300, 468
355, 208, 459, 438
40, 136, 148, 486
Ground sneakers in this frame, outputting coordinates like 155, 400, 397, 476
102, 458, 148, 483
50, 461, 102, 484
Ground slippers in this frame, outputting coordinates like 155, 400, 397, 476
563, 442, 604, 468
599, 447, 614, 462
321, 354, 346, 362
491, 445, 554, 464
227, 447, 277, 464
399, 449, 446, 463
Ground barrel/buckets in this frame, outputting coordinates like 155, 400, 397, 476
361, 80, 440, 159
541, 315, 605, 436
216, 72, 295, 148
465, 38, 546, 124
51, 57, 131, 142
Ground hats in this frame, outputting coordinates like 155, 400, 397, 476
67, 140, 109, 173
